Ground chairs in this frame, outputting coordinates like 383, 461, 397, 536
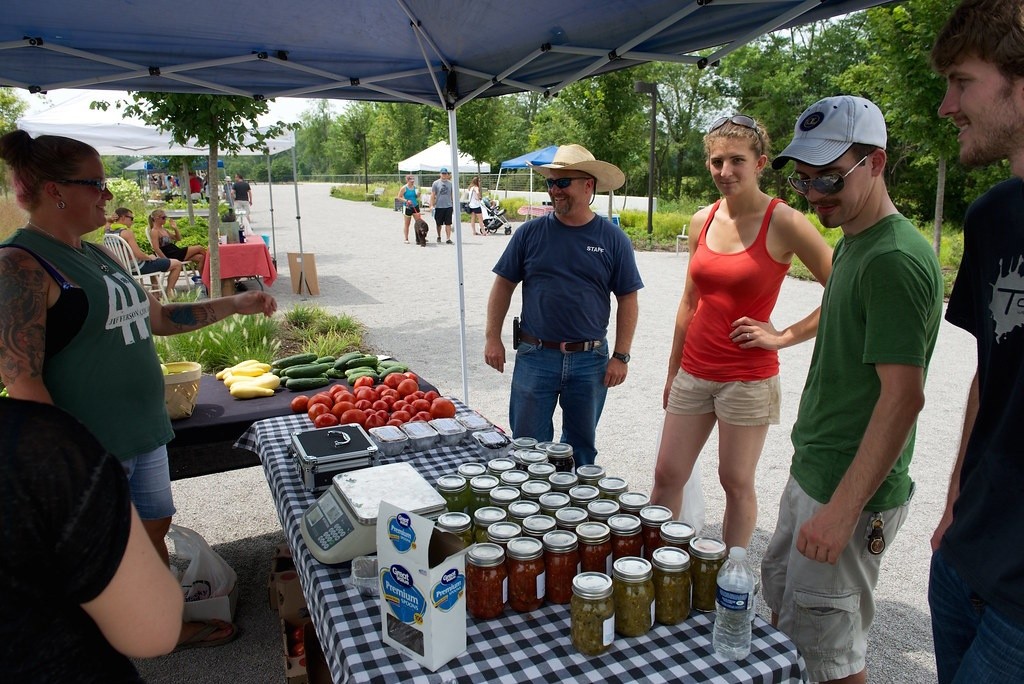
103, 234, 170, 304
145, 227, 198, 291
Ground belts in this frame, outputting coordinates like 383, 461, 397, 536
518, 332, 603, 352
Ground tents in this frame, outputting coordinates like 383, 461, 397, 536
492, 145, 560, 219
15, 90, 308, 301
398, 140, 491, 213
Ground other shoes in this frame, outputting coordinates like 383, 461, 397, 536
437, 237, 441, 242
405, 240, 410, 244
446, 239, 455, 245
473, 233, 481, 236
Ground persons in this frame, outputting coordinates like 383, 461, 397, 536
430, 168, 453, 245
398, 174, 422, 244
758, 96, 940, 684
0, 398, 184, 684
467, 177, 488, 236
484, 145, 645, 474
652, 113, 838, 562
0, 123, 277, 654
490, 201, 504, 215
927, 0, 1024, 684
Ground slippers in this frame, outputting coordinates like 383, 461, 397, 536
171, 619, 238, 653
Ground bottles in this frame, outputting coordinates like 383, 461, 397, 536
713, 546, 754, 661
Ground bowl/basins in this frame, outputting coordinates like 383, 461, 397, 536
369, 411, 512, 460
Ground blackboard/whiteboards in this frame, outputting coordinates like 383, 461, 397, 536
395, 198, 402, 210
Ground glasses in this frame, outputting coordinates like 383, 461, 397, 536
159, 216, 167, 219
788, 151, 873, 196
54, 178, 110, 192
709, 115, 765, 154
546, 177, 590, 189
125, 216, 133, 221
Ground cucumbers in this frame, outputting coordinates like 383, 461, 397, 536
271, 351, 407, 389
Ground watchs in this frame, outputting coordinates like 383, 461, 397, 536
612, 350, 631, 363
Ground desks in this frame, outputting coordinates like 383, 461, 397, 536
254, 398, 811, 684
202, 234, 278, 291
518, 205, 554, 222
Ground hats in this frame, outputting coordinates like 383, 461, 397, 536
772, 95, 887, 170
440, 168, 447, 174
526, 144, 625, 192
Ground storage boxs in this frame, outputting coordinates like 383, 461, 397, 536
270, 543, 334, 684
376, 499, 469, 672
171, 577, 239, 647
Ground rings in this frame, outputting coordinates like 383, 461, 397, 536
746, 333, 750, 340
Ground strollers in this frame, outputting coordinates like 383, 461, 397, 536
479, 196, 512, 235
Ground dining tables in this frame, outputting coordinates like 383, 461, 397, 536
0, 355, 439, 483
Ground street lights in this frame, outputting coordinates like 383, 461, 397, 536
355, 133, 369, 193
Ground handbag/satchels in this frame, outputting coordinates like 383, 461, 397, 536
465, 205, 471, 214
166, 523, 237, 602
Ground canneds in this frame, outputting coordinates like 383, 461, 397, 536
435, 438, 728, 657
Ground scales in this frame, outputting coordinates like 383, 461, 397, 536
300, 462, 450, 565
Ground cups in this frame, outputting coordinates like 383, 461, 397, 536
219, 235, 227, 245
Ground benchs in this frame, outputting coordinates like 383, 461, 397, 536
366, 188, 385, 202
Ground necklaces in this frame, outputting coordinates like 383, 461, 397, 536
27, 221, 110, 273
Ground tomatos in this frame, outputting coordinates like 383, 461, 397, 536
287, 626, 304, 658
290, 371, 456, 432
